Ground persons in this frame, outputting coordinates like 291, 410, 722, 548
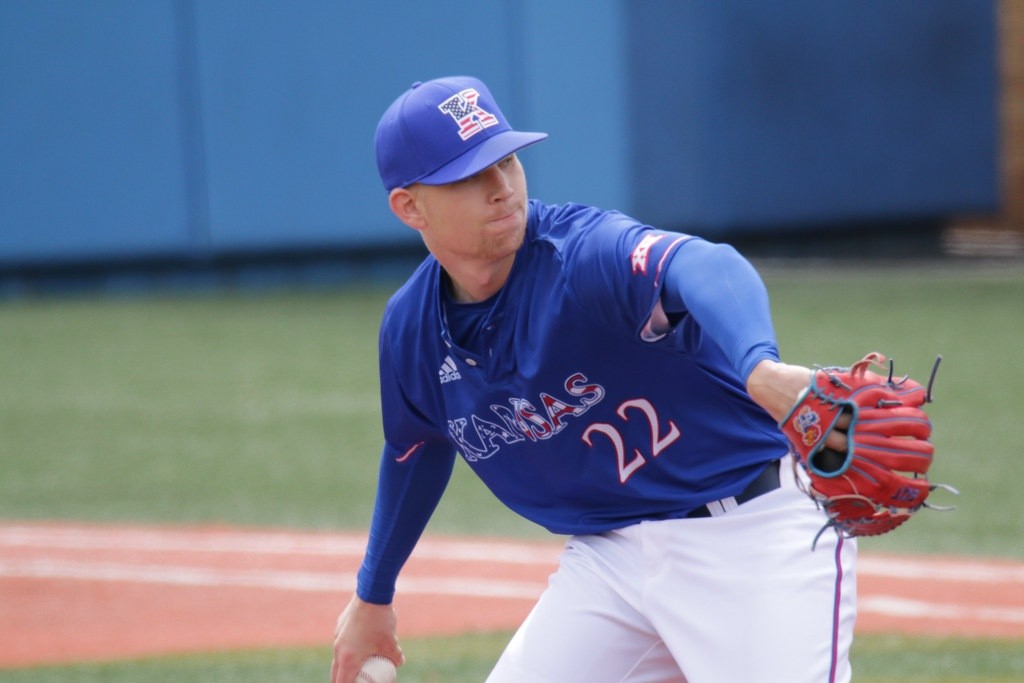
331, 74, 946, 683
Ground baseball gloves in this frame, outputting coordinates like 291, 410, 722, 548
778, 346, 962, 539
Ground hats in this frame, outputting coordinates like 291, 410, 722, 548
376, 74, 546, 193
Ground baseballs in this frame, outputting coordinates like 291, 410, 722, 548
351, 655, 400, 683
